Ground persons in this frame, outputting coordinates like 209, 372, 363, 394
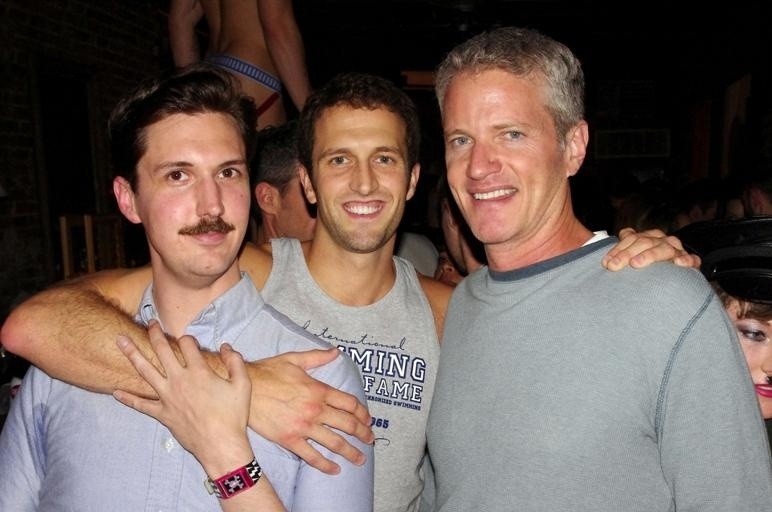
0, 1, 772, 511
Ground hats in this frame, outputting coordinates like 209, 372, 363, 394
679, 216, 772, 306
